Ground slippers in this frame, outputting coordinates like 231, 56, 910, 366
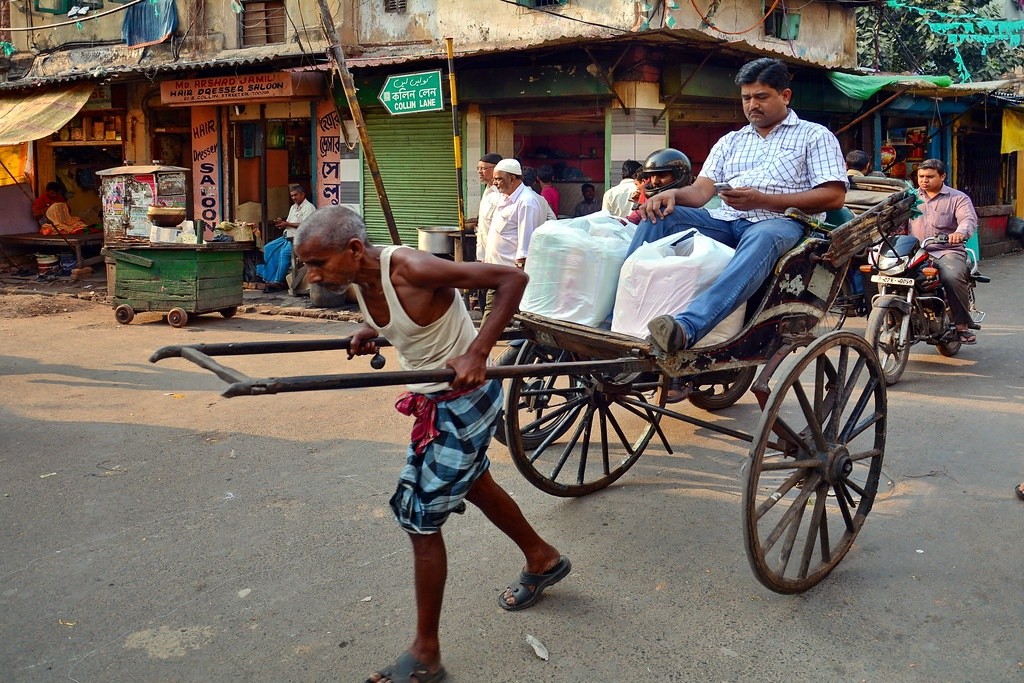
956, 330, 978, 344
879, 322, 896, 334
497, 554, 572, 611
363, 649, 446, 683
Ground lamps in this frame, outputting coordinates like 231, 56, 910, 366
233, 104, 245, 116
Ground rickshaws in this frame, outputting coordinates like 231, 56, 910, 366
149, 176, 925, 597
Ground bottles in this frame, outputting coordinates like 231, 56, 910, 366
271, 125, 285, 148
292, 157, 301, 176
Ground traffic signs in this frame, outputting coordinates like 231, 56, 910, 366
376, 67, 445, 117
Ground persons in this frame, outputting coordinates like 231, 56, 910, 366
32, 182, 71, 224
536, 165, 560, 219
293, 205, 572, 683
573, 183, 602, 217
475, 152, 558, 328
520, 166, 541, 195
619, 148, 693, 226
603, 159, 643, 217
880, 159, 978, 345
256, 184, 317, 294
624, 58, 850, 404
844, 150, 870, 177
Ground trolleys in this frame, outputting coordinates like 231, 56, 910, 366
95, 159, 258, 330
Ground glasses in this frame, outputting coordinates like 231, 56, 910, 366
477, 166, 494, 171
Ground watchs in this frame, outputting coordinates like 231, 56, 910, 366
514, 262, 525, 267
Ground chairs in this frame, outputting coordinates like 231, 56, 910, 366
511, 205, 859, 376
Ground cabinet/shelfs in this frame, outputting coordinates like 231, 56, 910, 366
47, 112, 122, 147
266, 147, 309, 223
512, 155, 606, 183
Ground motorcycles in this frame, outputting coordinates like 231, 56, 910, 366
856, 228, 992, 387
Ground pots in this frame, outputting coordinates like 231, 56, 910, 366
417, 226, 460, 253
147, 206, 187, 226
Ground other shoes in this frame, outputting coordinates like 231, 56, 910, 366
646, 314, 683, 354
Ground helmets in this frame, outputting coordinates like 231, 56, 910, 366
642, 147, 693, 199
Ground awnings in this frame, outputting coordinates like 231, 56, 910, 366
834, 76, 1024, 135
282, 28, 913, 126
0, 83, 101, 147
1, 41, 334, 89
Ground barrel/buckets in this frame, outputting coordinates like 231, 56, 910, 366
36, 254, 79, 276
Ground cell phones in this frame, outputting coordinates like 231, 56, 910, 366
714, 183, 734, 191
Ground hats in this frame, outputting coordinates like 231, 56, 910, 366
479, 152, 503, 165
494, 158, 522, 175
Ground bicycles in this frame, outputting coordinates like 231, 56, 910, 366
808, 247, 880, 338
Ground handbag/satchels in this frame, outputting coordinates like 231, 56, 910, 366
610, 228, 748, 350
517, 208, 638, 328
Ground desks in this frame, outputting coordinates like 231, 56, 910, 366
0, 231, 103, 269
